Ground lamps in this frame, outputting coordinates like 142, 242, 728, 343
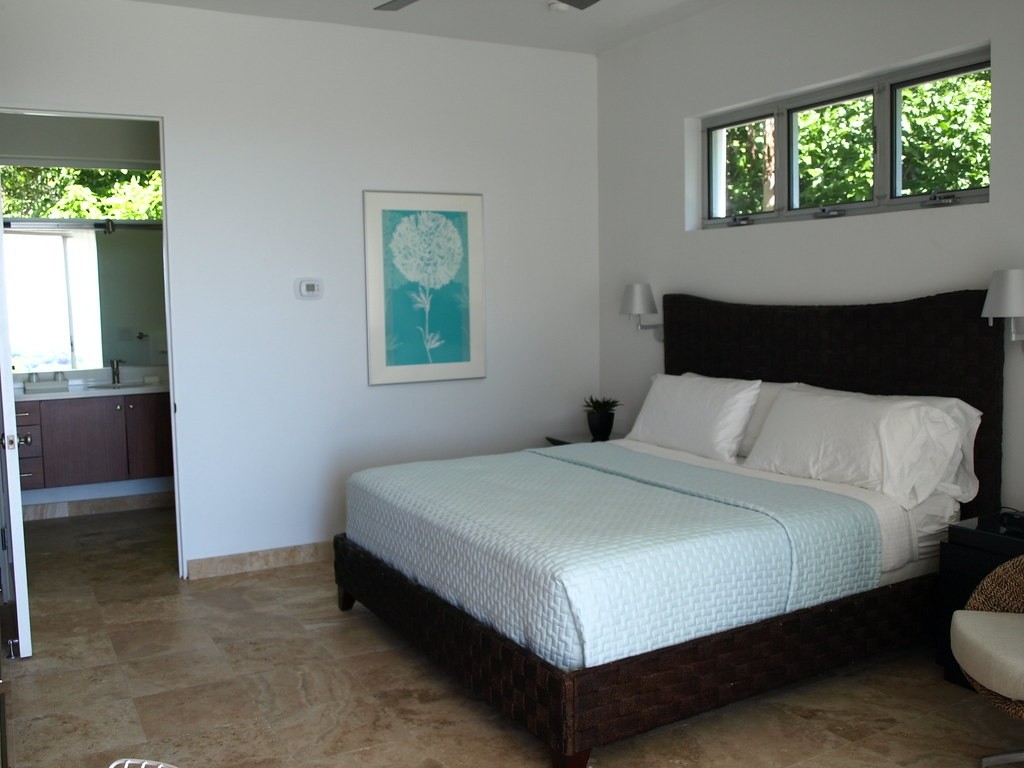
620, 284, 663, 341
981, 269, 1024, 352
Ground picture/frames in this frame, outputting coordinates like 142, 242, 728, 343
364, 191, 488, 386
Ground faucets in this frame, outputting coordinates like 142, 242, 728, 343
110, 359, 120, 384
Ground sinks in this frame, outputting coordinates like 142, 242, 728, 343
87, 383, 150, 389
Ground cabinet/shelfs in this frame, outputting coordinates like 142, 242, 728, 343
40, 392, 174, 488
15, 402, 45, 490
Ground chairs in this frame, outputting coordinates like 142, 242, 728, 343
961, 555, 1024, 768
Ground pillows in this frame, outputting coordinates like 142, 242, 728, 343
625, 373, 762, 463
680, 373, 797, 458
743, 386, 959, 512
795, 383, 986, 504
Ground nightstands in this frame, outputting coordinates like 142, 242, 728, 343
545, 431, 627, 446
940, 511, 1024, 692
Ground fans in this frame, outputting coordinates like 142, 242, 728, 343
374, 0, 599, 12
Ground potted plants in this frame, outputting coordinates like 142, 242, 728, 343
580, 395, 624, 438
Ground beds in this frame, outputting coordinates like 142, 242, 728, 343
332, 289, 1006, 768
